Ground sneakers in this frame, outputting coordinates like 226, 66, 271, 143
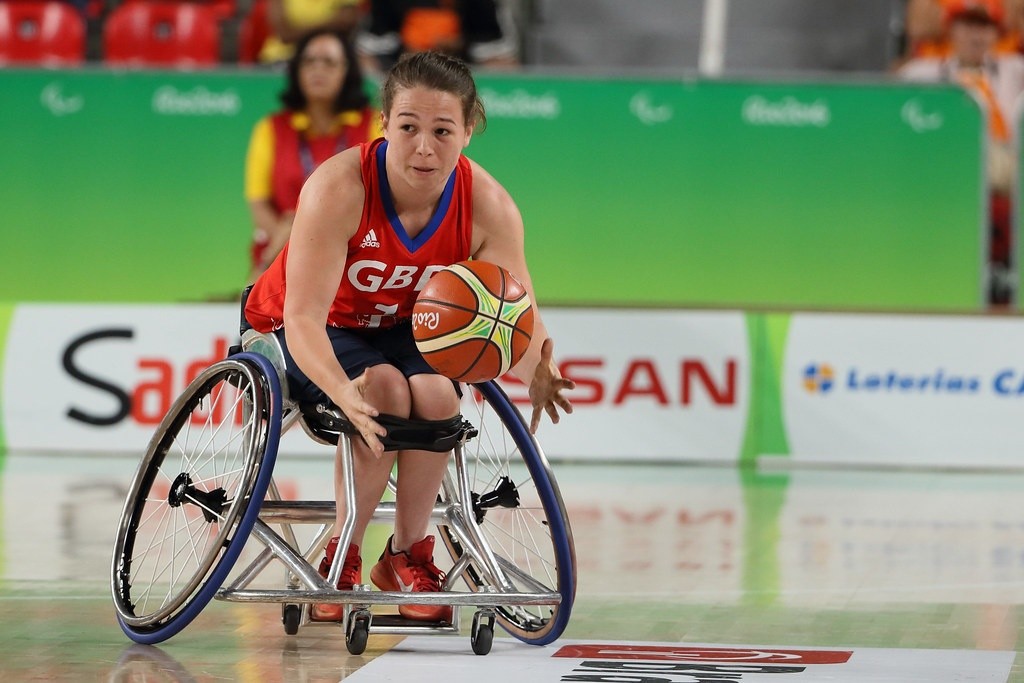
370, 534, 453, 623
310, 536, 362, 624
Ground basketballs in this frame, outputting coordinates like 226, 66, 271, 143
411, 261, 536, 382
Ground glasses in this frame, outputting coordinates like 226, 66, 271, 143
299, 56, 347, 69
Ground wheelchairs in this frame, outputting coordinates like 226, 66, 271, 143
109, 284, 580, 656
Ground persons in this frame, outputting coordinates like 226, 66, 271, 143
244, 0, 519, 288
893, 0, 1024, 305
241, 49, 576, 624
